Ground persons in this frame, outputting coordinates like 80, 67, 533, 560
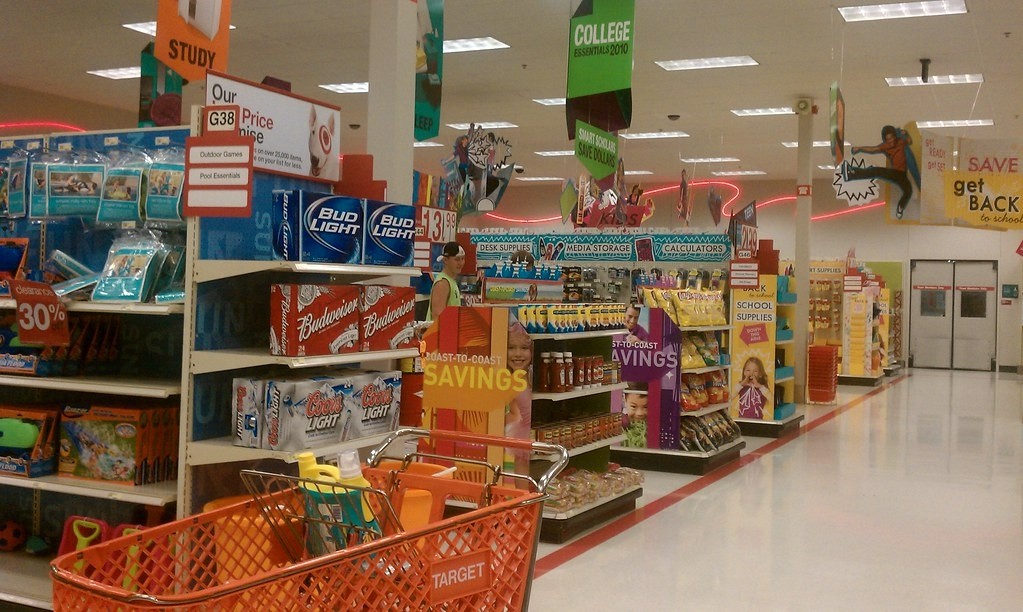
731, 357, 773, 418
626, 306, 651, 347
423, 242, 466, 327
507, 325, 532, 493
621, 392, 648, 422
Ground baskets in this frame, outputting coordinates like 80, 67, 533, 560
808, 345, 838, 402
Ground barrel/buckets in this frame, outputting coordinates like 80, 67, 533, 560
363, 463, 453, 554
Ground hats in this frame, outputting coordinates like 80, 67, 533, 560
436, 242, 465, 263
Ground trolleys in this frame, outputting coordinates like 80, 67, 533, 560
49, 428, 570, 612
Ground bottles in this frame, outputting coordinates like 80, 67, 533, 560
538, 351, 621, 393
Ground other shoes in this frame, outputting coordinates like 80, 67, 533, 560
896, 204, 904, 220
843, 160, 854, 181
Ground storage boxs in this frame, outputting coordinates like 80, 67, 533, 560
0, 190, 414, 611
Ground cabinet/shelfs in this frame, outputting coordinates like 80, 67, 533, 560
422, 260, 643, 544
777, 247, 885, 387
455, 200, 803, 439
854, 248, 902, 377
608, 285, 746, 476
0, 68, 422, 612
